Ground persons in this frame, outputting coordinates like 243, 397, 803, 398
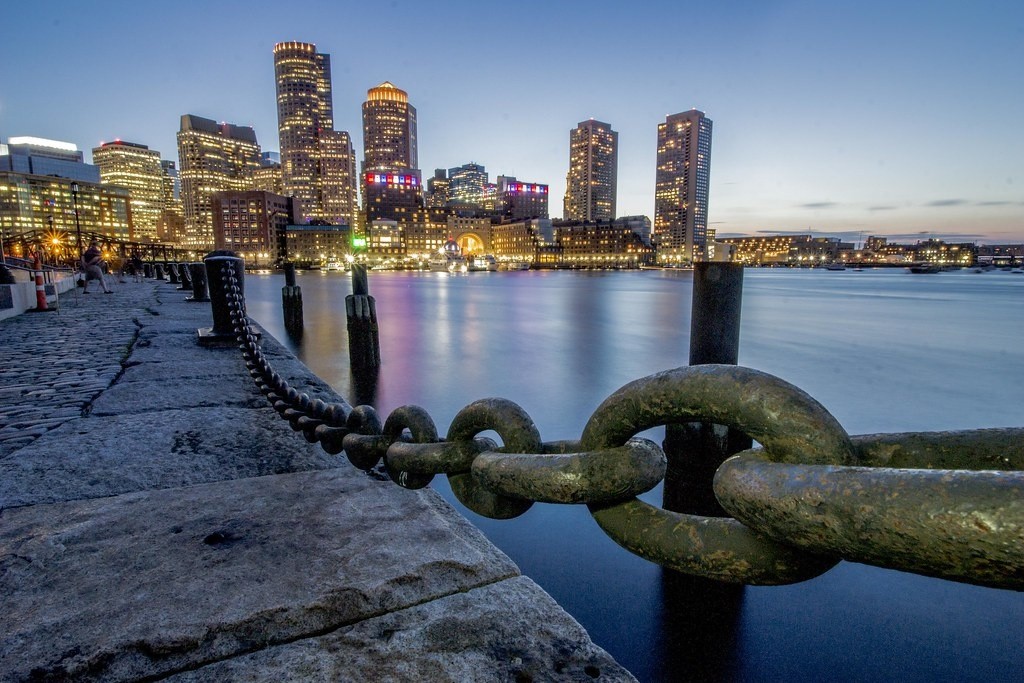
131, 255, 143, 282
82, 241, 115, 294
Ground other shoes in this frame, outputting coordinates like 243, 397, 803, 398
105, 290, 114, 294
83, 291, 90, 294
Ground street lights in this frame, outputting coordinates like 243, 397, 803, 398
71, 181, 84, 270
47, 215, 54, 230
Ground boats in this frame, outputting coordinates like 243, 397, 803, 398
910, 264, 940, 273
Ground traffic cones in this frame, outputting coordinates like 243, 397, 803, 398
24, 260, 57, 312
